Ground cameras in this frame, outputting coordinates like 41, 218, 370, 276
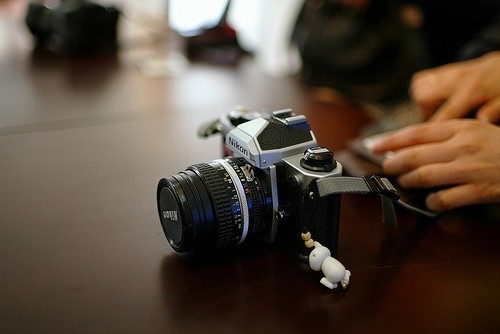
155, 104, 344, 271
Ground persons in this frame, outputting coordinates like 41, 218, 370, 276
288, 1, 500, 215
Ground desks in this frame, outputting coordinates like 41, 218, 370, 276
0, 1, 500, 333
